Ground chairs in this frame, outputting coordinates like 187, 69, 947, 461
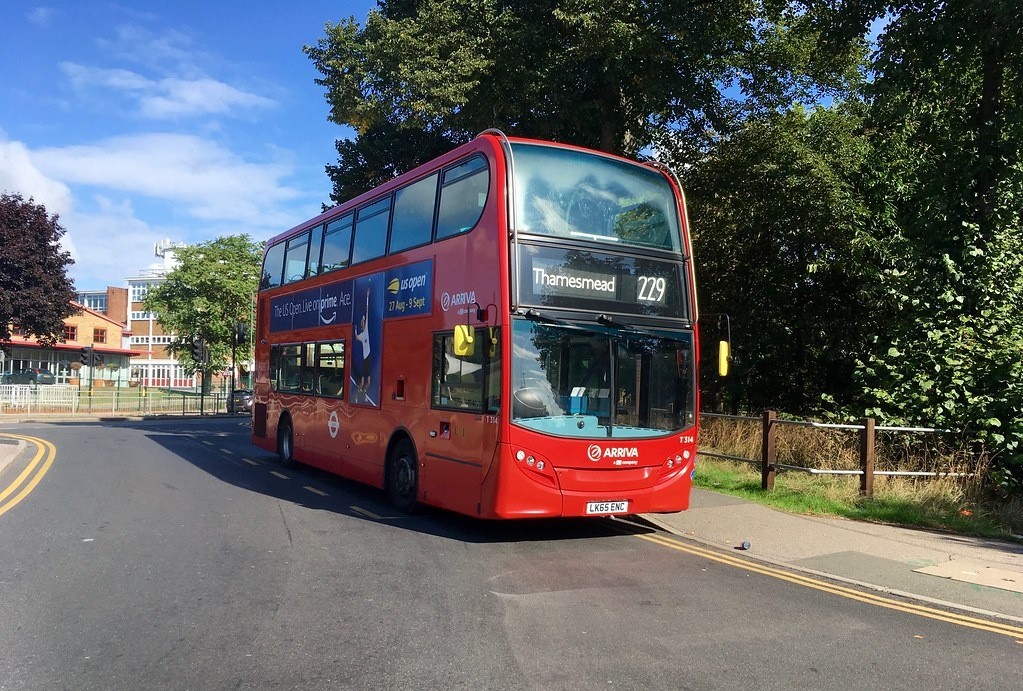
270, 363, 344, 398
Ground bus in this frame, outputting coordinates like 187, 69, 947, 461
250, 129, 734, 521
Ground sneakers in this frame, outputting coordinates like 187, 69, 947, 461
353, 388, 360, 403
362, 388, 367, 401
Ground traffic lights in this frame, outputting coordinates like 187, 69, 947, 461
80, 347, 91, 366
93, 353, 101, 366
191, 339, 204, 362
238, 322, 247, 343
201, 348, 210, 363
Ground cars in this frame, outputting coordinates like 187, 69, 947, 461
7, 368, 55, 384
226, 388, 253, 415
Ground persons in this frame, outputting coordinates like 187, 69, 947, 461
354, 289, 372, 403
